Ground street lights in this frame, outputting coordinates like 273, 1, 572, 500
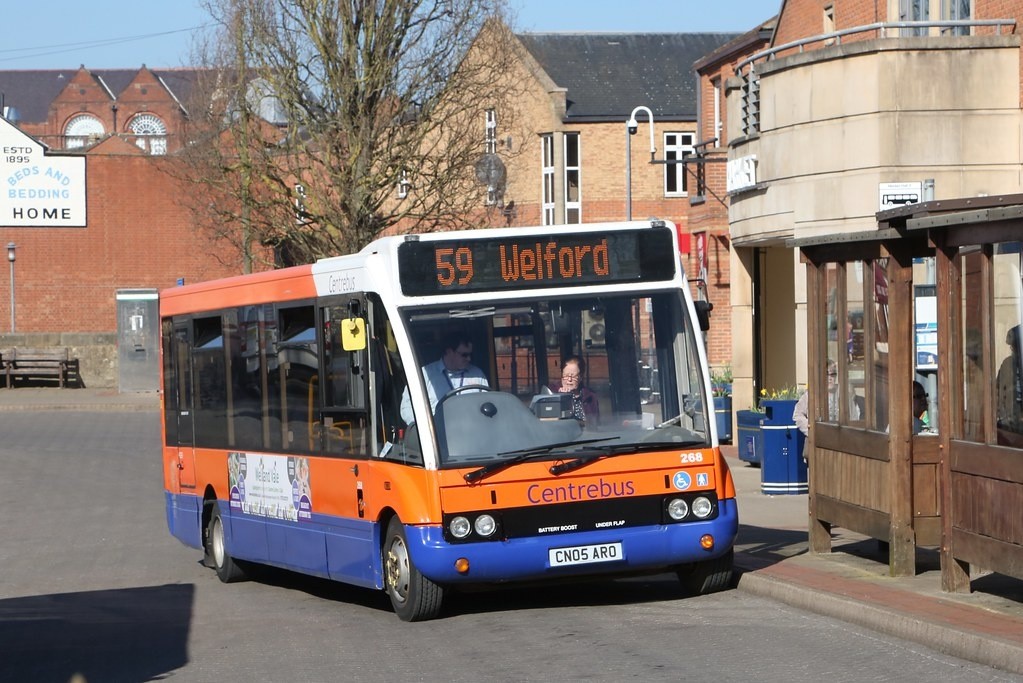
6, 243, 19, 332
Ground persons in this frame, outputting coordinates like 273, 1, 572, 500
399, 333, 489, 425
545, 355, 601, 430
994, 325, 1023, 433
791, 361, 860, 484
885, 380, 933, 436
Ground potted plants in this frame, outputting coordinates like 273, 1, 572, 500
686, 373, 732, 443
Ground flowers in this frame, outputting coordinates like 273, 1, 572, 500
760, 383, 809, 399
721, 360, 726, 365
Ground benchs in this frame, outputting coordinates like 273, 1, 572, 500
0, 347, 68, 388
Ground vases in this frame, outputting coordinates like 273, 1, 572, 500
736, 409, 767, 468
712, 383, 732, 395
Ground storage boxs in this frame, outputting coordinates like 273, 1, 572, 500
759, 399, 809, 496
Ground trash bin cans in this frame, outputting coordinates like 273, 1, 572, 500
756, 398, 809, 496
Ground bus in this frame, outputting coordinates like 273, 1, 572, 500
154, 220, 739, 626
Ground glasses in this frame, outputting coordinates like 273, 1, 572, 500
455, 350, 473, 358
828, 372, 836, 377
560, 371, 581, 379
911, 392, 930, 400
